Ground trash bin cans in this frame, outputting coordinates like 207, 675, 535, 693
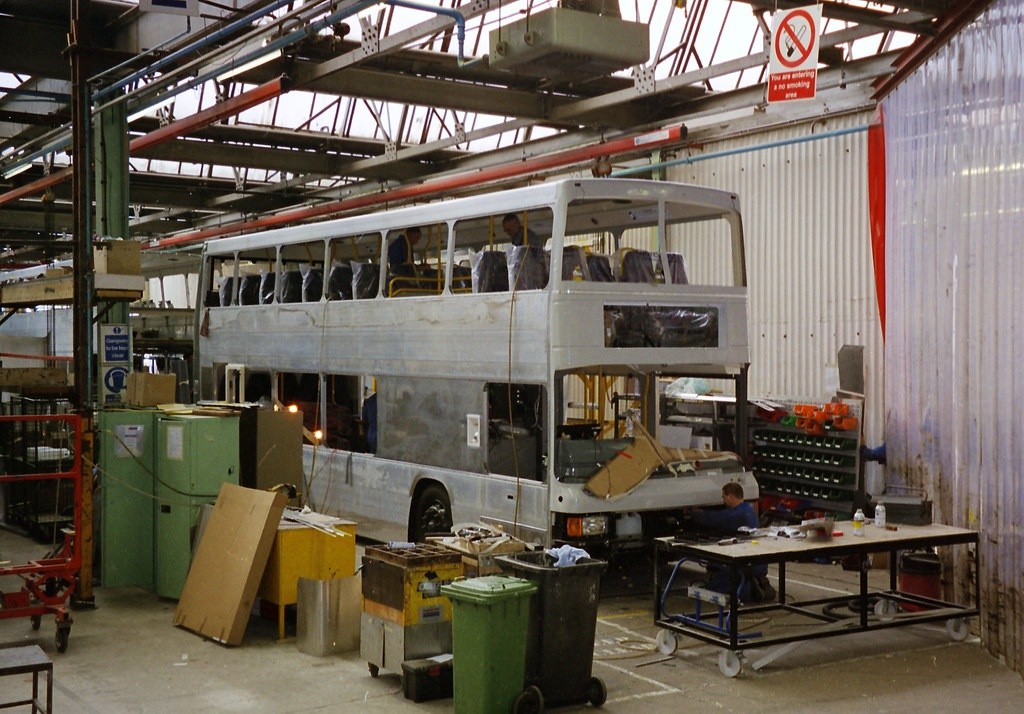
495, 551, 607, 712
440, 576, 538, 714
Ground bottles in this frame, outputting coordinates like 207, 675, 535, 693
875, 500, 886, 528
853, 509, 866, 537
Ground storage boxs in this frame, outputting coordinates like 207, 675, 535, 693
172, 482, 288, 646
93, 240, 141, 275
127, 373, 177, 406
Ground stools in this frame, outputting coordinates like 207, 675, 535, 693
0, 644, 54, 714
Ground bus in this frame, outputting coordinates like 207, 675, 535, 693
195, 176, 760, 556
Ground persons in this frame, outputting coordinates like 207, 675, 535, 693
387, 226, 431, 278
693, 483, 768, 595
502, 213, 541, 248
862, 431, 887, 467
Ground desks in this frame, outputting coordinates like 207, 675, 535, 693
653, 518, 981, 678
257, 508, 358, 641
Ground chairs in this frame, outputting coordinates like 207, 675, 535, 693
218, 246, 688, 347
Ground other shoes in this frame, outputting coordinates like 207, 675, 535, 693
751, 575, 777, 601
